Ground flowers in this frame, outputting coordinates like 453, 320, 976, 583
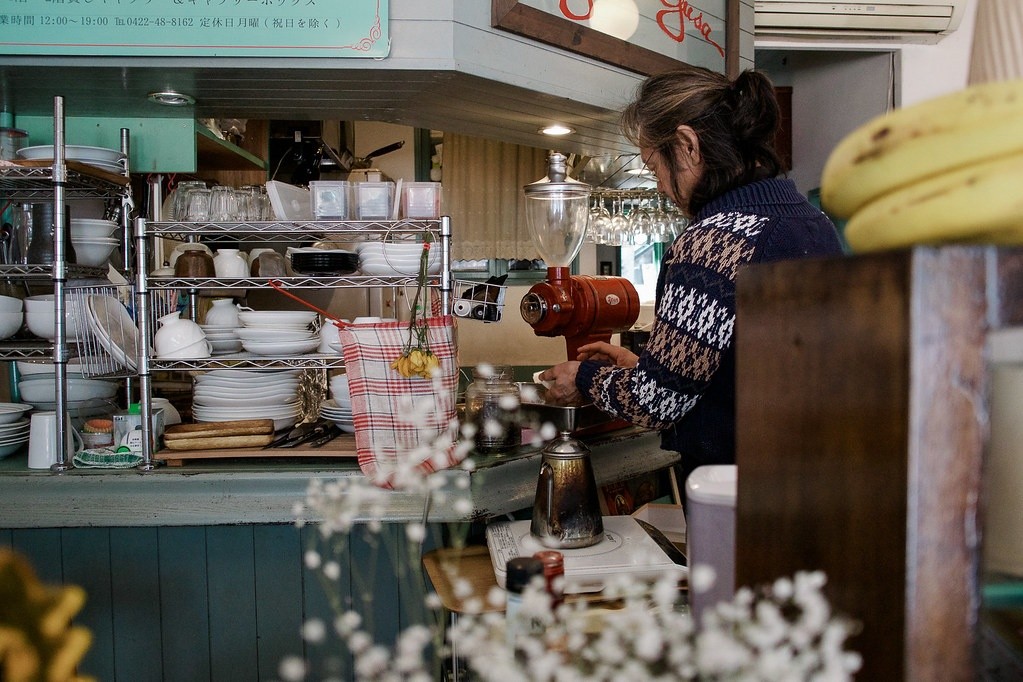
388, 229, 443, 380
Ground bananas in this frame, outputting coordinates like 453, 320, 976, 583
819, 79, 1023, 256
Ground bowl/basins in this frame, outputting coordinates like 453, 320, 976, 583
155, 310, 206, 355
0, 294, 94, 344
168, 243, 287, 278
17, 356, 120, 432
70, 217, 120, 267
318, 373, 355, 433
156, 339, 211, 358
317, 318, 350, 353
401, 181, 443, 218
352, 180, 395, 219
352, 316, 382, 323
308, 180, 351, 221
204, 299, 243, 325
151, 398, 181, 425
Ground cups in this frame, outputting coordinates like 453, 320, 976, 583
11, 202, 71, 264
28, 412, 84, 469
172, 180, 271, 222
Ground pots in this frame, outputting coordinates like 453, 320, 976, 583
353, 140, 405, 169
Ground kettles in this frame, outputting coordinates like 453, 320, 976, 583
530, 430, 604, 548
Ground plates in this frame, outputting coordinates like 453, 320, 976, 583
17, 144, 128, 175
291, 242, 442, 275
85, 295, 139, 373
199, 310, 322, 356
328, 342, 343, 352
0, 402, 33, 459
163, 370, 304, 452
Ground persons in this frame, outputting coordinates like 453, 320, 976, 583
537, 66, 843, 521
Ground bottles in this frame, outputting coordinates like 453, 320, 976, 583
534, 550, 565, 609
464, 365, 520, 456
505, 558, 543, 601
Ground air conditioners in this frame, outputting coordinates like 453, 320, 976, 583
754, 0, 966, 44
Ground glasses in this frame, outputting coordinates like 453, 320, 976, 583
637, 137, 662, 184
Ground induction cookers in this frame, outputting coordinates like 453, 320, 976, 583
485, 514, 689, 590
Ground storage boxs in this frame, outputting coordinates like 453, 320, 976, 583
401, 180, 442, 220
354, 181, 395, 220
307, 181, 352, 220
629, 503, 687, 554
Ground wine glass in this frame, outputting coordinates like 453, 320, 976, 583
582, 186, 687, 246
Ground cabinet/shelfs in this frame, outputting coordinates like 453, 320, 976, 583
0, 96, 454, 477
